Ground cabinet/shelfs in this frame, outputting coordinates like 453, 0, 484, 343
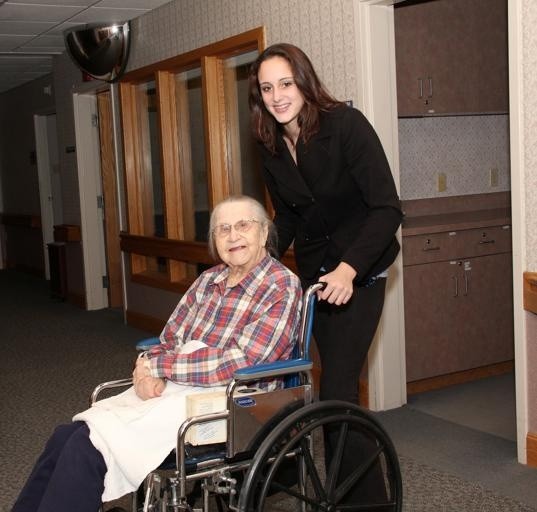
394, 2, 508, 118
401, 224, 515, 383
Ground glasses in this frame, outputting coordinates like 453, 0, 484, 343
211, 220, 261, 239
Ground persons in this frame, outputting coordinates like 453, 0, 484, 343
241, 41, 408, 511
6, 192, 304, 511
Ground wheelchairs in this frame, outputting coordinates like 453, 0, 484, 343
89, 281, 402, 510
